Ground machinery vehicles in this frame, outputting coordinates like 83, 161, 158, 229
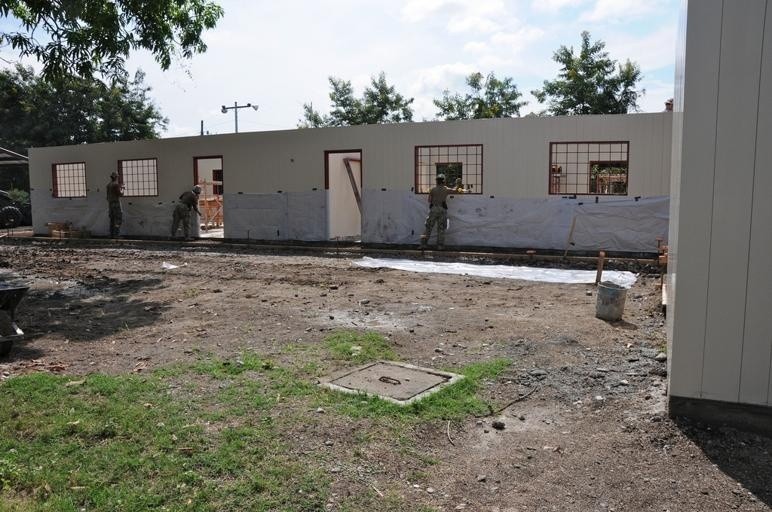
0, 190, 32, 227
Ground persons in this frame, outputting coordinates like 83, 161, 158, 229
452, 178, 464, 190
171, 184, 202, 241
106, 172, 125, 239
418, 173, 461, 251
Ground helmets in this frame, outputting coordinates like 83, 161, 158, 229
192, 185, 201, 194
110, 172, 120, 177
436, 174, 445, 183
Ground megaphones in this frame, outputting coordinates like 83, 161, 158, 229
220, 105, 228, 113
249, 104, 259, 111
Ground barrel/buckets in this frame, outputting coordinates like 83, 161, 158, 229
595, 280, 628, 322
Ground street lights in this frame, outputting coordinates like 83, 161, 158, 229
220, 102, 261, 133
